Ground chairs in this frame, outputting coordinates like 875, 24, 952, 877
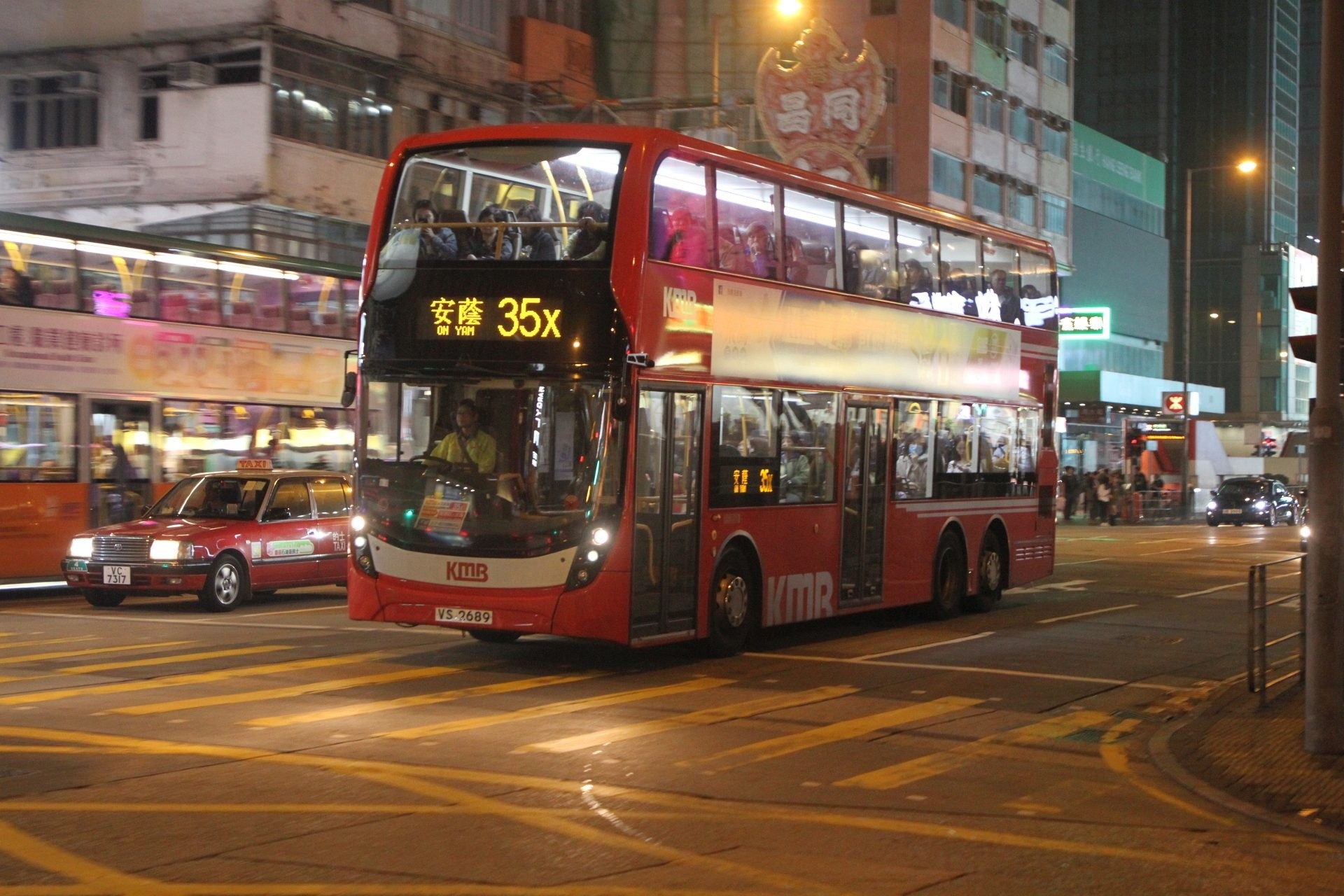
439, 210, 1043, 325
650, 430, 1009, 512
28, 279, 358, 339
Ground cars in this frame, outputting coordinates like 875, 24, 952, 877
1285, 483, 1310, 521
1205, 476, 1299, 527
61, 452, 357, 613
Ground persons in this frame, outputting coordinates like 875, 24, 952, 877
200, 480, 231, 516
1056, 462, 1164, 529
0, 267, 35, 307
413, 192, 1051, 329
647, 414, 1037, 500
111, 442, 137, 480
423, 398, 497, 478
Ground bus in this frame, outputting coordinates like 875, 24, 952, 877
340, 121, 1061, 667
0, 242, 427, 594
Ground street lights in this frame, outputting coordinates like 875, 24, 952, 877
1180, 157, 1258, 519
712, 0, 802, 136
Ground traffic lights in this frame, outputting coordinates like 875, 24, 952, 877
1126, 431, 1147, 458
1285, 283, 1344, 408
1262, 437, 1276, 457
1251, 445, 1260, 457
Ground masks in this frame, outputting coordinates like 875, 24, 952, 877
908, 444, 924, 456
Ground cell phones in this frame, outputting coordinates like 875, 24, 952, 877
419, 219, 429, 225
